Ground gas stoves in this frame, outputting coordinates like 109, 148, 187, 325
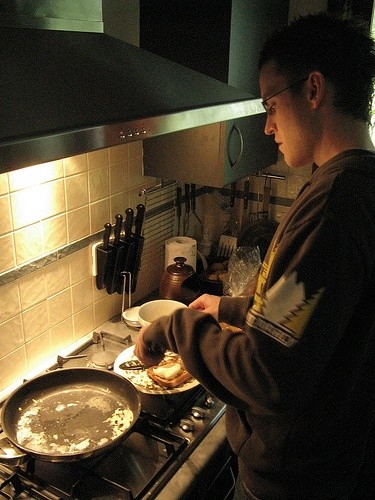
0, 331, 238, 500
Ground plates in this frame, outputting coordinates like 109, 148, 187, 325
113, 344, 201, 395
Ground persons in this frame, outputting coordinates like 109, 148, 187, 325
134, 15, 375, 500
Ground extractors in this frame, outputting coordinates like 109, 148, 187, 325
0, 0, 267, 175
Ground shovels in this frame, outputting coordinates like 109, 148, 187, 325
119, 357, 148, 372
216, 178, 240, 261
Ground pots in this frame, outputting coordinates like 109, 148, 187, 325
0, 367, 142, 463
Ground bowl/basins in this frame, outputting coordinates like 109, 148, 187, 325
138, 299, 187, 327
196, 256, 229, 296
123, 306, 140, 328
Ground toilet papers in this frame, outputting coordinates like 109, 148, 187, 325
164, 236, 197, 272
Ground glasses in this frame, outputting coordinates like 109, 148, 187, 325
261, 77, 309, 113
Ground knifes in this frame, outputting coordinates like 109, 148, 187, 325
184, 183, 190, 234
103, 204, 146, 249
229, 181, 236, 226
177, 187, 182, 236
241, 181, 250, 231
